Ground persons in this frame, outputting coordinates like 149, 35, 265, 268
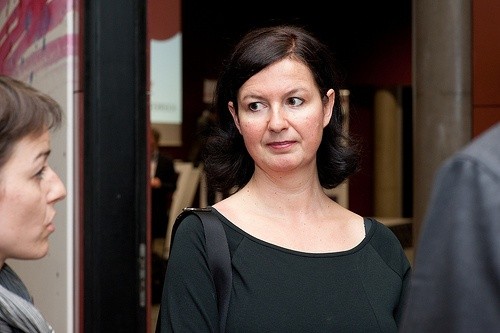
154, 28, 413, 332
0, 74, 67, 333
151, 128, 175, 239
395, 124, 500, 333
